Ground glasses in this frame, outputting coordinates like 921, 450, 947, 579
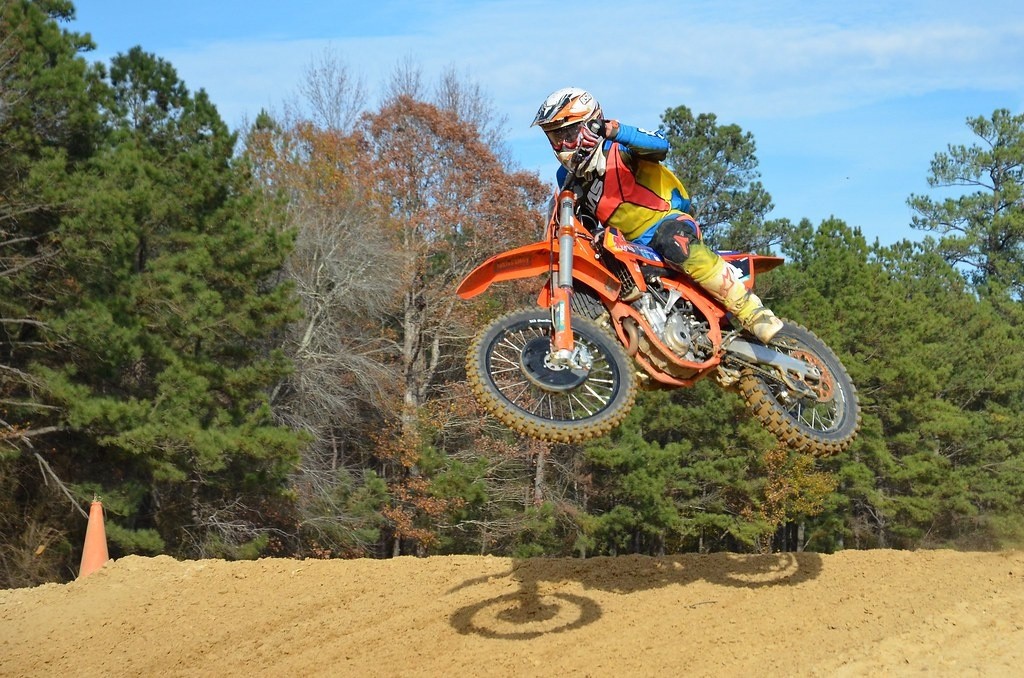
545, 102, 602, 150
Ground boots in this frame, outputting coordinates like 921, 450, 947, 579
634, 369, 680, 392
693, 255, 784, 343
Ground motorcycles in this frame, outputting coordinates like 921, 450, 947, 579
454, 123, 862, 458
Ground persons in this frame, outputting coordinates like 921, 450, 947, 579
532, 87, 785, 344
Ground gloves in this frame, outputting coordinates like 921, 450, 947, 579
577, 118, 607, 154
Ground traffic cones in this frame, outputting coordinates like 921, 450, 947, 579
78, 502, 109, 577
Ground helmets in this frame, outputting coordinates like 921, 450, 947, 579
531, 87, 605, 176
